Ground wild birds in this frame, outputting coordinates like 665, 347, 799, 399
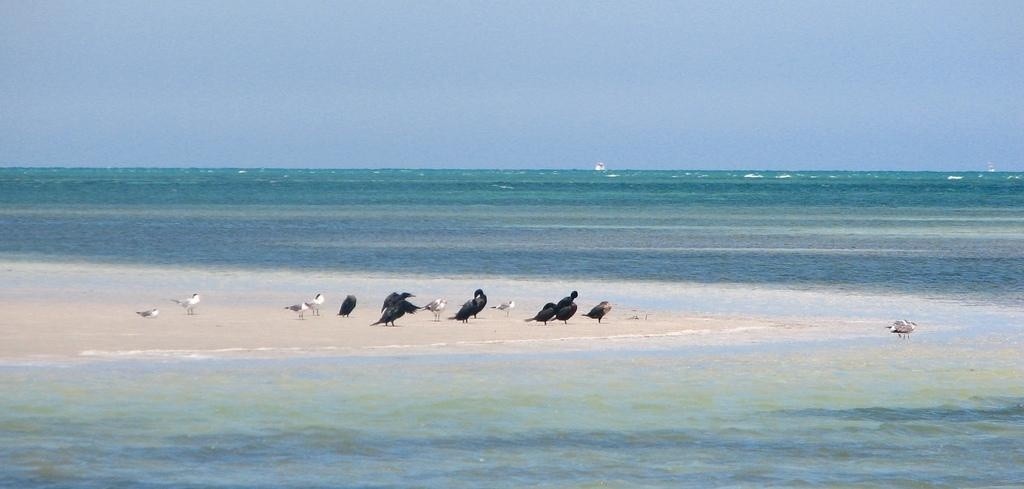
370, 292, 424, 326
305, 293, 325, 317
285, 302, 309, 320
422, 298, 447, 322
337, 295, 356, 319
524, 291, 578, 325
490, 301, 514, 318
172, 293, 201, 316
470, 289, 487, 319
449, 298, 479, 323
886, 319, 917, 339
135, 308, 159, 319
582, 301, 613, 323
